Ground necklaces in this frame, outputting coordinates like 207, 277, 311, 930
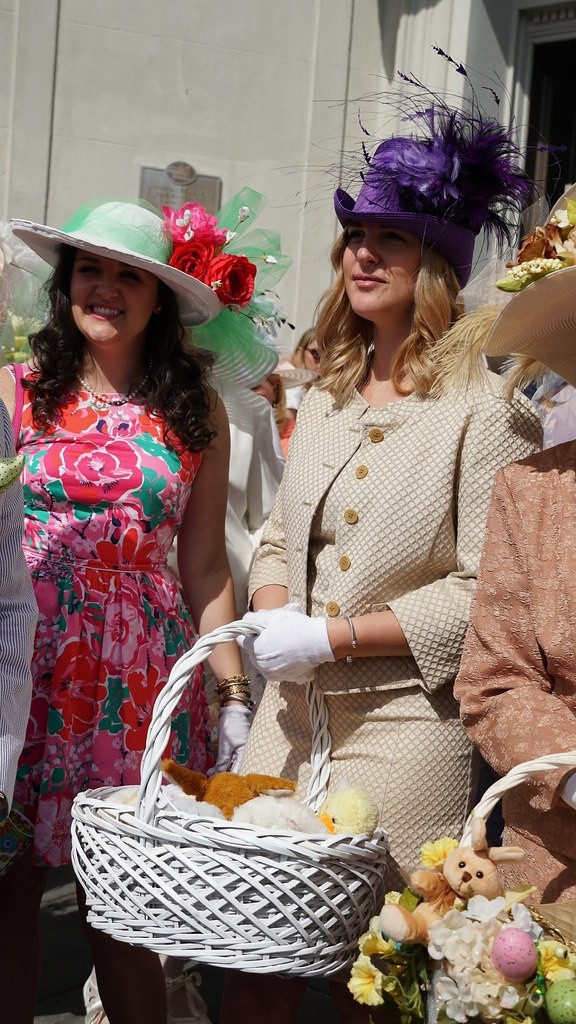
76, 372, 149, 406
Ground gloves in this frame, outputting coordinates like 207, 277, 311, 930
236, 603, 337, 685
206, 705, 253, 776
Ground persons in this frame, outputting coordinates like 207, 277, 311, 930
0, 402, 37, 820
276, 328, 323, 419
212, 378, 285, 613
233, 137, 545, 1024
451, 177, 576, 950
0, 197, 256, 1024
251, 373, 296, 458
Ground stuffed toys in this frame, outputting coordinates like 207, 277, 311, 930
162, 761, 295, 822
230, 796, 332, 835
381, 816, 527, 945
104, 782, 228, 821
316, 787, 380, 835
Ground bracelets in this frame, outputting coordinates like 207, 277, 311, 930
215, 674, 256, 711
344, 615, 358, 663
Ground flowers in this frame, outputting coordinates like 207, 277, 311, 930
158, 199, 297, 341
388, 140, 464, 202
345, 836, 576, 1024
495, 197, 576, 292
4, 309, 46, 364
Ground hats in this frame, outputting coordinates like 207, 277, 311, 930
324, 40, 564, 289
426, 185, 576, 403
193, 327, 279, 393
272, 360, 316, 389
10, 186, 292, 383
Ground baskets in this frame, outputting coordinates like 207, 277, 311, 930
71, 621, 390, 977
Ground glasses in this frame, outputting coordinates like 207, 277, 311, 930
306, 348, 321, 359
